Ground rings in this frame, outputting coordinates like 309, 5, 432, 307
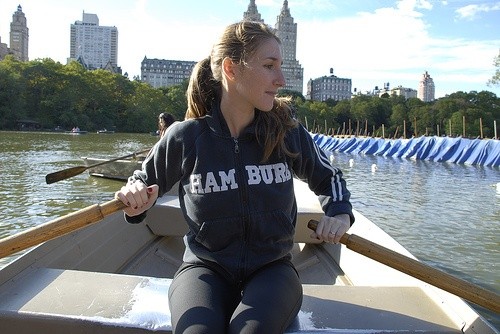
329, 232, 336, 235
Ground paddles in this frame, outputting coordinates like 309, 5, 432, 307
297, 219, 500, 314
0, 191, 150, 259
46, 148, 151, 184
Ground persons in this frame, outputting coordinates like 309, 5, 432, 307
114, 20, 351, 334
156, 112, 177, 141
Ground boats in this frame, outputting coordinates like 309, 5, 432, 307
98, 130, 116, 134
10, 120, 59, 131
151, 131, 160, 135
59, 130, 86, 134
80, 155, 145, 181
